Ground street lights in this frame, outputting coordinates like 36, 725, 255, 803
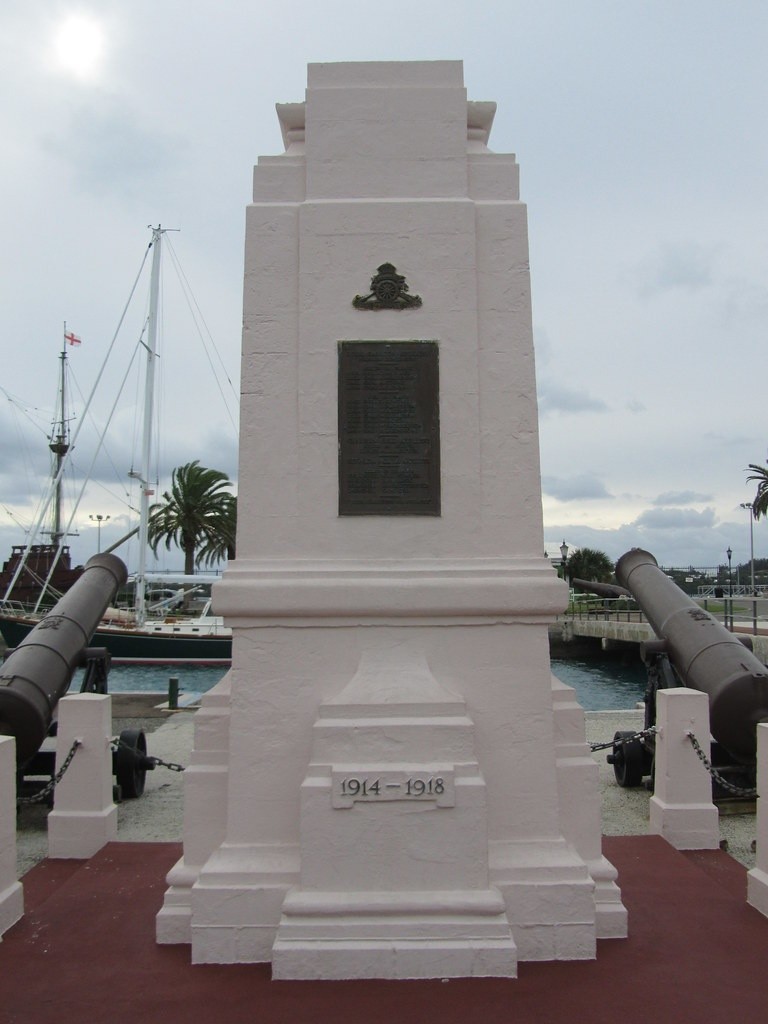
726, 546, 735, 635
559, 538, 569, 580
87, 514, 110, 552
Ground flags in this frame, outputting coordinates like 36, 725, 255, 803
65, 331, 82, 347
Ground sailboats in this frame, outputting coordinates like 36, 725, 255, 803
0, 224, 236, 670
